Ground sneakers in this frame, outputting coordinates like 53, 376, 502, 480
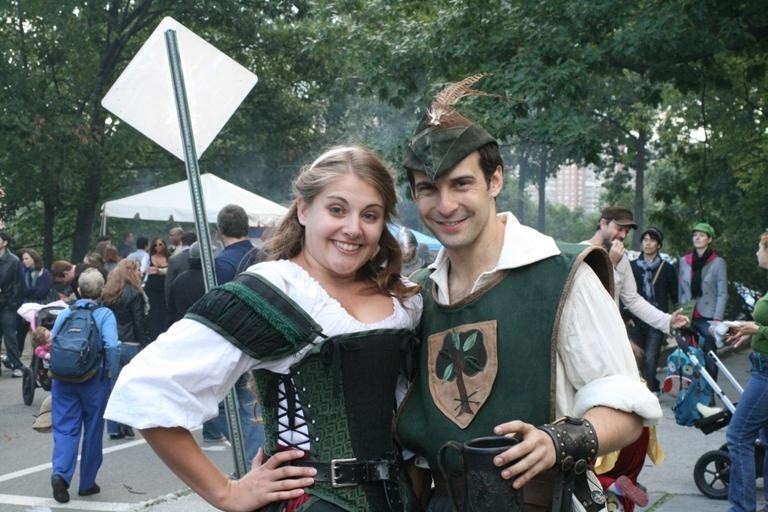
1, 355, 25, 378
52, 475, 71, 503
77, 484, 100, 497
615, 476, 649, 508
109, 424, 229, 447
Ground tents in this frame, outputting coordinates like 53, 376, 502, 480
386, 222, 441, 251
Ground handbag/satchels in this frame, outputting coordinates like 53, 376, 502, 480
662, 344, 705, 399
672, 375, 717, 427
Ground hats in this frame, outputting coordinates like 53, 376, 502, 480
600, 206, 638, 230
400, 72, 500, 180
692, 222, 715, 240
642, 226, 664, 249
31, 394, 54, 433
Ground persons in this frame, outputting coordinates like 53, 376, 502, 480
397, 227, 436, 278
388, 73, 663, 512
577, 206, 767, 512
104, 148, 423, 512
2, 204, 267, 503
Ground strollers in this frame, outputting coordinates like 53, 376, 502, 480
671, 313, 768, 499
22, 304, 69, 406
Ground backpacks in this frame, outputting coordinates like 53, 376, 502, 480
50, 302, 106, 385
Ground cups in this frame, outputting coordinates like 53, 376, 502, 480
435, 433, 523, 511
